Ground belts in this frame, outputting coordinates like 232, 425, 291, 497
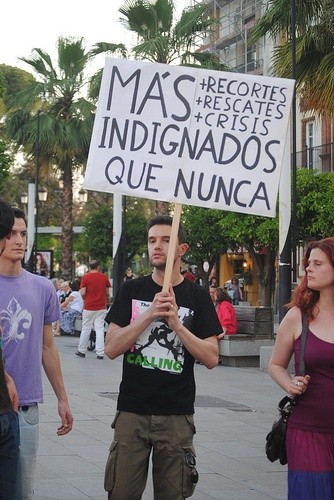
18, 405, 28, 411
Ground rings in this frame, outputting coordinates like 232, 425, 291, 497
295, 381, 300, 386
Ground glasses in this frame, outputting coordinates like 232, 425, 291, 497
186, 451, 199, 485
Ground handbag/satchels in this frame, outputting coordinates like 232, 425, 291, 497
265, 396, 293, 465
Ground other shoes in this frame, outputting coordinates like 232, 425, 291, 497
75, 351, 85, 357
97, 356, 103, 359
52, 330, 60, 337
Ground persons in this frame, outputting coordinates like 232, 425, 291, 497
75, 261, 112, 359
30, 253, 139, 337
268, 236, 334, 500
0, 198, 75, 499
104, 215, 225, 499
179, 268, 244, 366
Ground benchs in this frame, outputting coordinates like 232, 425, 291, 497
223, 307, 255, 340
